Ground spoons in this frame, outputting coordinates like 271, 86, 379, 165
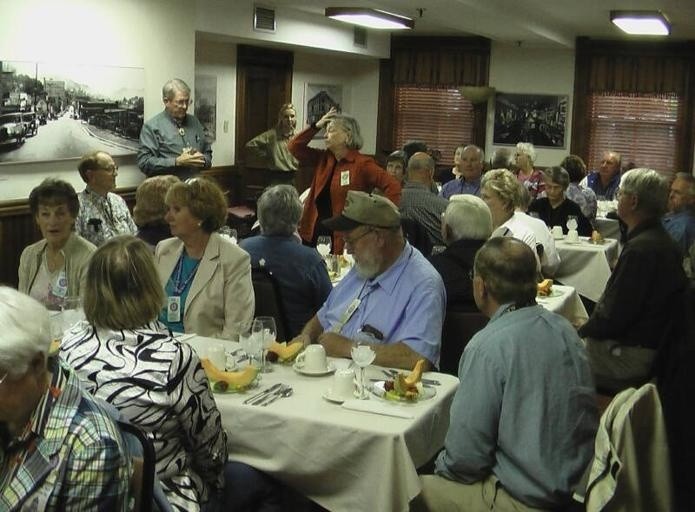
261, 388, 294, 407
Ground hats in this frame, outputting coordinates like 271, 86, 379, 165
322, 190, 401, 232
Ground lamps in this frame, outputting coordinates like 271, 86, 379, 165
325, 8, 414, 32
611, 10, 671, 36
457, 81, 496, 146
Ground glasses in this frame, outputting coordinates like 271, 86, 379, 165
184, 176, 198, 198
468, 268, 486, 290
614, 187, 641, 204
164, 96, 193, 106
342, 227, 375, 248
97, 164, 118, 175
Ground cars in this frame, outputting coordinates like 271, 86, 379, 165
1, 110, 40, 148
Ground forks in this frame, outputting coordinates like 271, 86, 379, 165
251, 384, 289, 406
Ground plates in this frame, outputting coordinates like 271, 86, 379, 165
564, 240, 582, 244
292, 363, 338, 376
320, 389, 370, 403
367, 379, 439, 405
554, 237, 566, 240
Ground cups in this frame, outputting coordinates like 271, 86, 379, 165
550, 226, 563, 237
203, 343, 226, 373
295, 343, 329, 371
333, 367, 364, 399
567, 230, 580, 240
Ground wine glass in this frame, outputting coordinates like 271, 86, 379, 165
343, 242, 356, 267
350, 332, 378, 399
238, 320, 264, 380
252, 315, 278, 374
316, 235, 332, 259
62, 295, 81, 327
565, 215, 579, 230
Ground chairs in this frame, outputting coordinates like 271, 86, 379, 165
106, 411, 160, 510
594, 280, 694, 404
248, 266, 287, 344
576, 386, 673, 511
438, 311, 489, 377
402, 221, 432, 258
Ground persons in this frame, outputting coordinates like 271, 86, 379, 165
0, 151, 257, 342
56, 232, 229, 511
238, 184, 332, 343
245, 103, 300, 171
301, 190, 448, 373
288, 107, 401, 255
413, 236, 602, 510
386, 150, 408, 178
0, 286, 134, 512
402, 142, 694, 390
137, 79, 212, 176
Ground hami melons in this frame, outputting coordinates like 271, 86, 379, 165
394, 360, 425, 392
537, 278, 552, 290
592, 230, 602, 241
268, 343, 303, 361
202, 360, 259, 386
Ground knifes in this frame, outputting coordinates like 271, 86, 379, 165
245, 383, 280, 404
